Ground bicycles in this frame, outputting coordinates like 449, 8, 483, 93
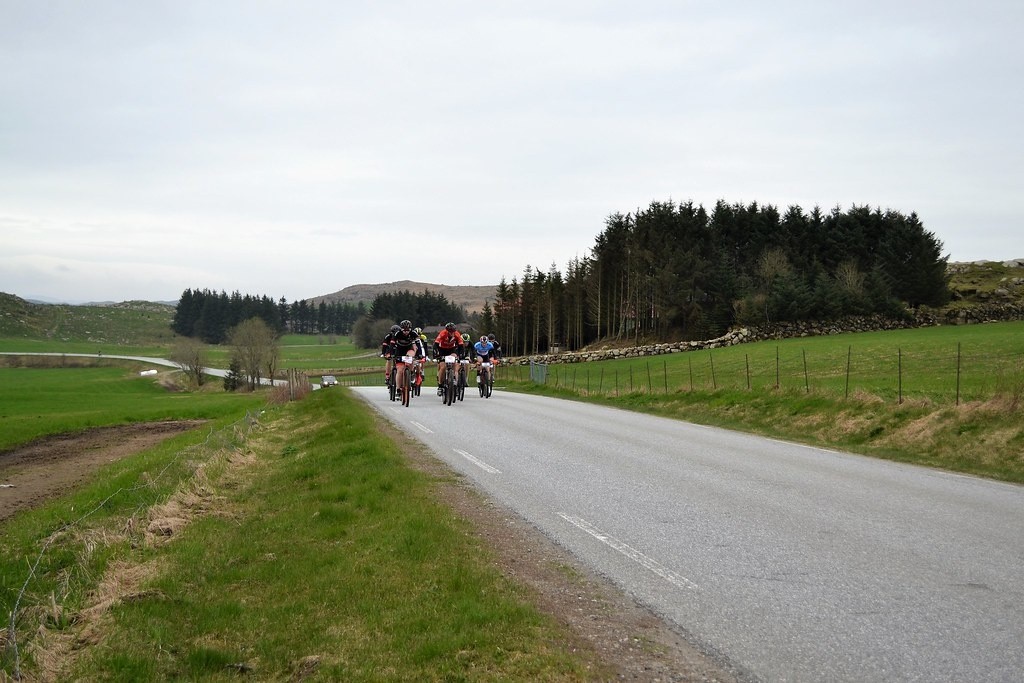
433, 356, 502, 406
380, 355, 430, 407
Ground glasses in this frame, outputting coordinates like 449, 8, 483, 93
403, 330, 409, 331
481, 341, 486, 344
489, 340, 494, 342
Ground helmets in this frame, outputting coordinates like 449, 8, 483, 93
414, 327, 422, 335
488, 334, 496, 340
480, 336, 488, 342
391, 325, 401, 331
445, 323, 457, 332
400, 320, 412, 328
461, 334, 470, 342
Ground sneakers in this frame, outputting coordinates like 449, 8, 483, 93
476, 376, 480, 383
492, 375, 495, 383
437, 385, 442, 396
453, 379, 458, 386
421, 374, 425, 380
395, 389, 401, 400
411, 375, 416, 386
466, 378, 470, 387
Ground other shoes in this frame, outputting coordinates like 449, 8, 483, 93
385, 374, 390, 383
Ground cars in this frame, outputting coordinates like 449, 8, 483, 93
320, 375, 339, 389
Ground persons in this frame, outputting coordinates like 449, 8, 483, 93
98, 350, 101, 356
433, 322, 501, 396
380, 320, 430, 398
